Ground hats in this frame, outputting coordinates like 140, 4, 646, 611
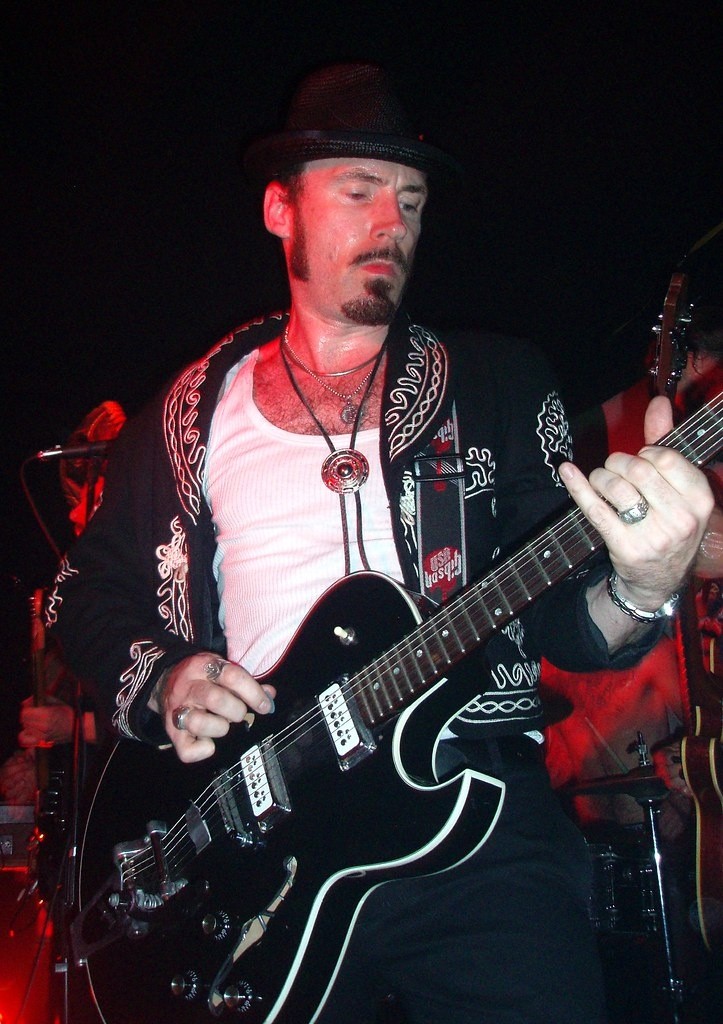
249, 62, 465, 179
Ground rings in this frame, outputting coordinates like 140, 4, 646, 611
617, 502, 648, 524
172, 705, 193, 730
203, 657, 232, 685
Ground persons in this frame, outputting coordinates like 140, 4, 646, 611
39, 60, 716, 1024
541, 283, 723, 1024
0, 399, 127, 896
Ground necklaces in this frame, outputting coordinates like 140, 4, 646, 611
280, 322, 390, 576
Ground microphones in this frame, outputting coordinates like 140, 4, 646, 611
34, 438, 116, 462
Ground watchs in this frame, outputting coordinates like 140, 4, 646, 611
606, 569, 691, 624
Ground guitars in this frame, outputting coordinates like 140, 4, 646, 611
645, 269, 723, 953
63, 388, 723, 1022
